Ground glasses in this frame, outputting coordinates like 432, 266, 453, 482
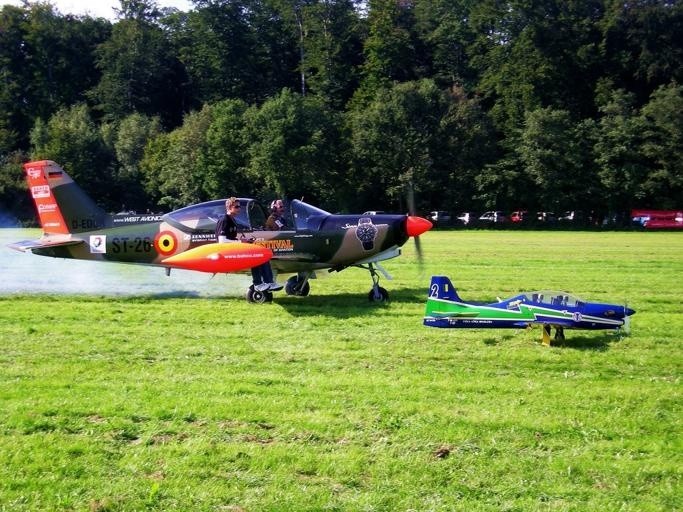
231, 205, 241, 208
277, 206, 284, 208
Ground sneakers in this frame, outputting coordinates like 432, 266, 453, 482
253, 282, 284, 292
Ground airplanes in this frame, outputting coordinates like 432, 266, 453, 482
2, 156, 436, 305
420, 275, 637, 345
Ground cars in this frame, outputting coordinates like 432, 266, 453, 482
510, 211, 529, 223
478, 212, 507, 224
629, 207, 683, 230
454, 212, 477, 227
430, 211, 451, 222
558, 210, 585, 222
536, 212, 557, 222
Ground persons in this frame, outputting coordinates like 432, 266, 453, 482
215, 198, 284, 294
262, 197, 288, 231
